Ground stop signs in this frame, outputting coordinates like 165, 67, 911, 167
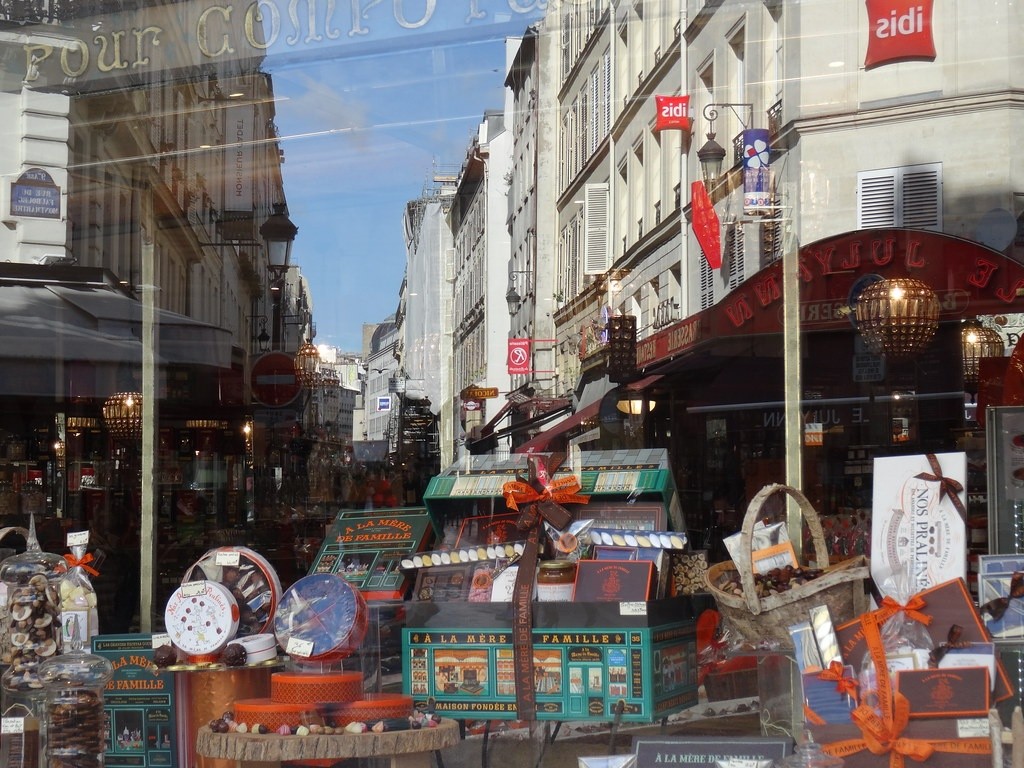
249, 349, 304, 409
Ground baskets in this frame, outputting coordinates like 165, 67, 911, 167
703, 484, 871, 651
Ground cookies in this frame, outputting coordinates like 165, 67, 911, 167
0, 571, 103, 768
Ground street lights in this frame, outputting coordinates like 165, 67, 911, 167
258, 202, 301, 351
